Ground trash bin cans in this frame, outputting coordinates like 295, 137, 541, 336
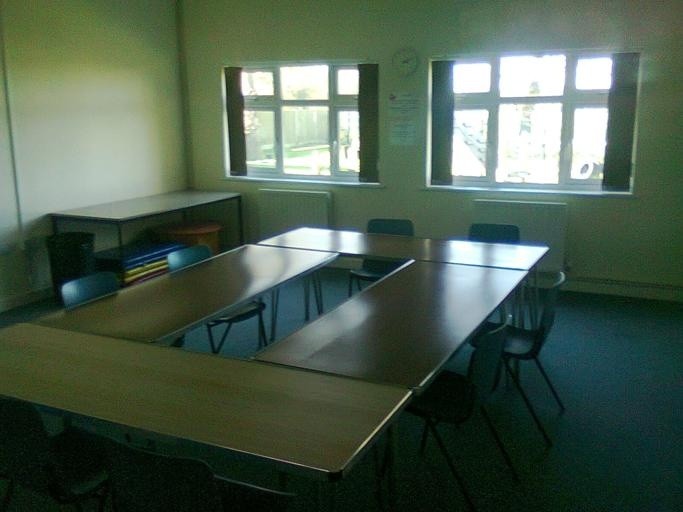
46, 232, 94, 304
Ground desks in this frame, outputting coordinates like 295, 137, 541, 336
48, 188, 243, 288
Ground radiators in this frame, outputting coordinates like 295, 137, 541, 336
257, 188, 329, 238
473, 199, 568, 271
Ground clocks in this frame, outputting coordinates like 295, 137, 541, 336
391, 49, 417, 75
